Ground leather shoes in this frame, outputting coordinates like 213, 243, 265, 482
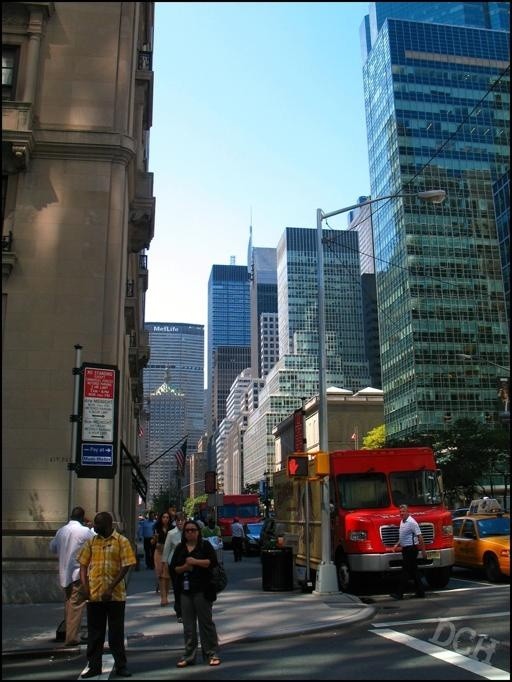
114, 665, 133, 677
81, 667, 103, 679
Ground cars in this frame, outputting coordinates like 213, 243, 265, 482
243, 522, 264, 558
453, 497, 512, 586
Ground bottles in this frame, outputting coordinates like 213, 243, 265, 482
183, 571, 190, 591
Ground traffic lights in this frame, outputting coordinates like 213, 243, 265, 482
288, 455, 309, 477
317, 453, 331, 476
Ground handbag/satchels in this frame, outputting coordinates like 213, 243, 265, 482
209, 558, 228, 593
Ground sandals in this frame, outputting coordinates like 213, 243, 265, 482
209, 652, 221, 666
175, 657, 197, 667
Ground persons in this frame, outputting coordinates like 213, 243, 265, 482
85, 517, 98, 535
76, 511, 137, 677
390, 503, 428, 599
160, 512, 187, 622
138, 510, 247, 570
49, 506, 95, 648
168, 520, 221, 667
150, 511, 174, 608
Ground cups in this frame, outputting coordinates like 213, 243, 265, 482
277, 537, 285, 547
153, 534, 159, 543
270, 540, 277, 548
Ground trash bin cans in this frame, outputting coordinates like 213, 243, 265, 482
262, 546, 294, 591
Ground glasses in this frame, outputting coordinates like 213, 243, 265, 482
183, 527, 199, 533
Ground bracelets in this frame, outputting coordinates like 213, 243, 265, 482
394, 545, 397, 547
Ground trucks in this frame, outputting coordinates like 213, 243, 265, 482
206, 494, 261, 551
270, 444, 457, 593
192, 502, 208, 527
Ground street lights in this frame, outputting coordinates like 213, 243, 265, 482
456, 353, 510, 372
313, 187, 447, 595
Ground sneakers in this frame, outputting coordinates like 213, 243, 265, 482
412, 592, 426, 598
389, 592, 405, 601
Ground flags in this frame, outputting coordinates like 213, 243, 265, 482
137, 424, 144, 439
173, 438, 187, 473
352, 433, 360, 441
